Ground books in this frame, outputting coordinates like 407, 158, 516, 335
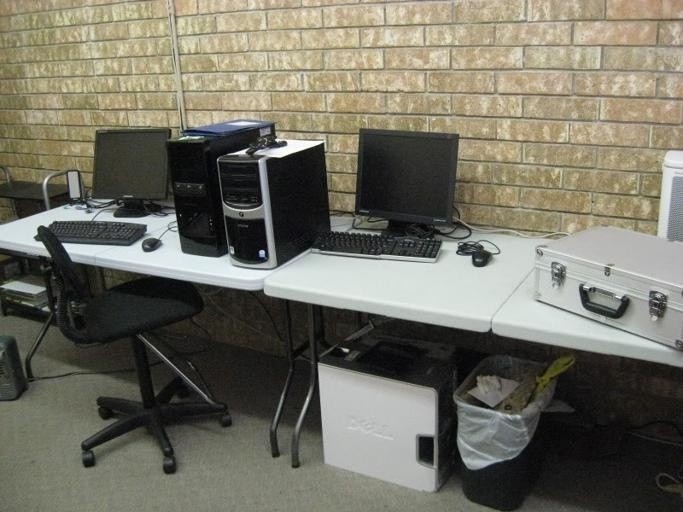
179, 117, 271, 138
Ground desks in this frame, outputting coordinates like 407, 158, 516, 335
259, 209, 556, 473
0, 193, 363, 460
490, 265, 681, 371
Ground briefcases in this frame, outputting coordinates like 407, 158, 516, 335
535, 225, 683, 351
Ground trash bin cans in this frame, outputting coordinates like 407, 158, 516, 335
453, 355, 557, 511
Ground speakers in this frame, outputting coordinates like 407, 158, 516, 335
66, 170, 86, 205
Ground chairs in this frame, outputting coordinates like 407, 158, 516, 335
33, 223, 233, 476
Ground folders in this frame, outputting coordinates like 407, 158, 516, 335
182, 119, 275, 137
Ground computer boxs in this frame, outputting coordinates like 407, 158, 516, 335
166, 119, 277, 257
216, 139, 331, 269
316, 340, 459, 494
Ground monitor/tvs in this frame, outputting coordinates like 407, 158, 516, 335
354, 128, 460, 240
92, 127, 172, 218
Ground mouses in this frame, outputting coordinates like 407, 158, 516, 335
142, 238, 161, 252
472, 248, 490, 267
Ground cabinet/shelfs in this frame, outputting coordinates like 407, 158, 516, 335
0, 163, 91, 328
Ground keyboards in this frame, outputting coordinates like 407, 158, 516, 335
35, 221, 147, 246
311, 230, 444, 263
